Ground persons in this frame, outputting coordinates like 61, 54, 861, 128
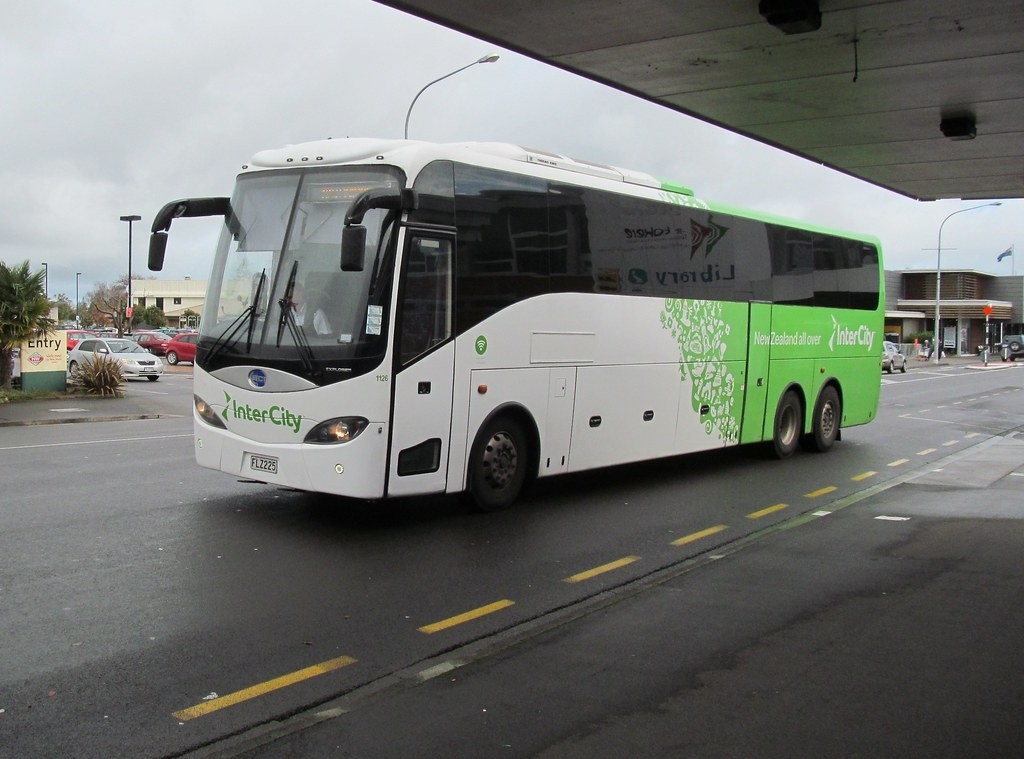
284, 282, 332, 334
927, 328, 942, 361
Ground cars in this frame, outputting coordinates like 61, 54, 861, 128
138, 327, 198, 339
63, 322, 118, 337
881, 340, 909, 374
68, 338, 165, 380
66, 330, 100, 350
165, 331, 197, 366
132, 332, 174, 356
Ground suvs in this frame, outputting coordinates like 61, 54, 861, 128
999, 334, 1024, 362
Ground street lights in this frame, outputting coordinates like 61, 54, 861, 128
404, 53, 500, 139
42, 262, 47, 300
932, 200, 1003, 364
119, 214, 142, 336
76, 272, 82, 330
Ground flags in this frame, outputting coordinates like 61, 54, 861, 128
997, 243, 1014, 262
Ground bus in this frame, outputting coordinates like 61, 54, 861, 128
144, 137, 887, 513
